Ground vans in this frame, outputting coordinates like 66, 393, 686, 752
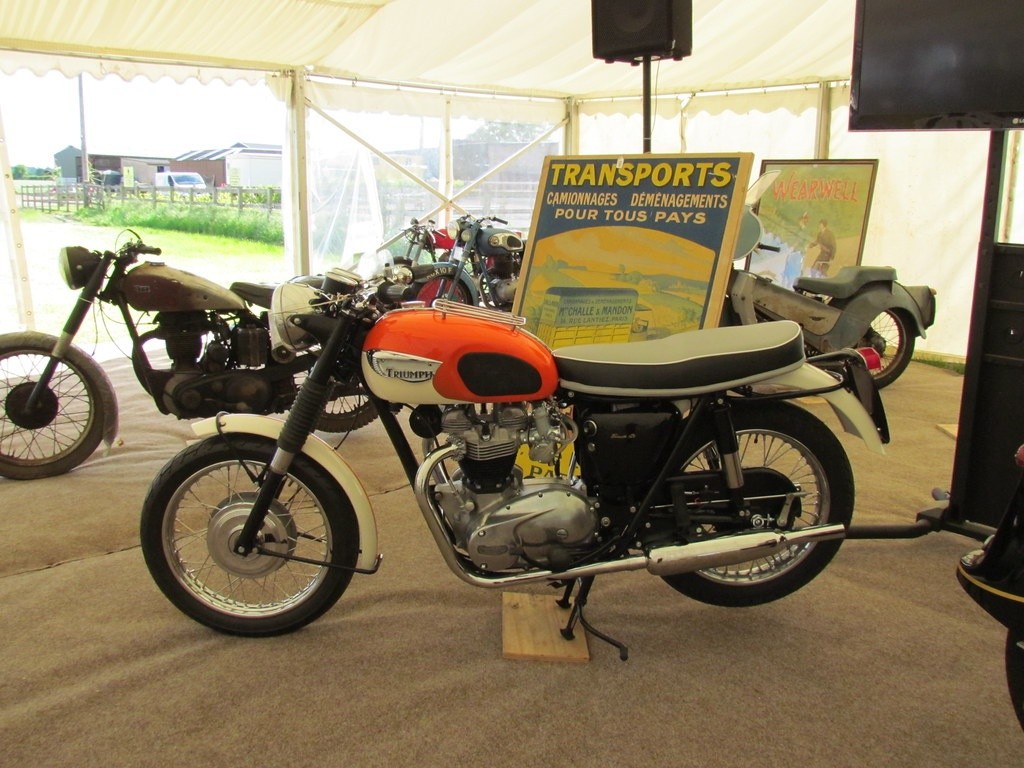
155, 172, 206, 192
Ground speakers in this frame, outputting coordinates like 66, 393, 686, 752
591, 0, 693, 66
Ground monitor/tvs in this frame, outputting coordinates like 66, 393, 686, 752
850, 0, 1024, 132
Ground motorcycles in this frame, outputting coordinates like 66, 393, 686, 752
717, 243, 936, 389
0, 229, 400, 480
392, 216, 528, 312
139, 267, 890, 637
